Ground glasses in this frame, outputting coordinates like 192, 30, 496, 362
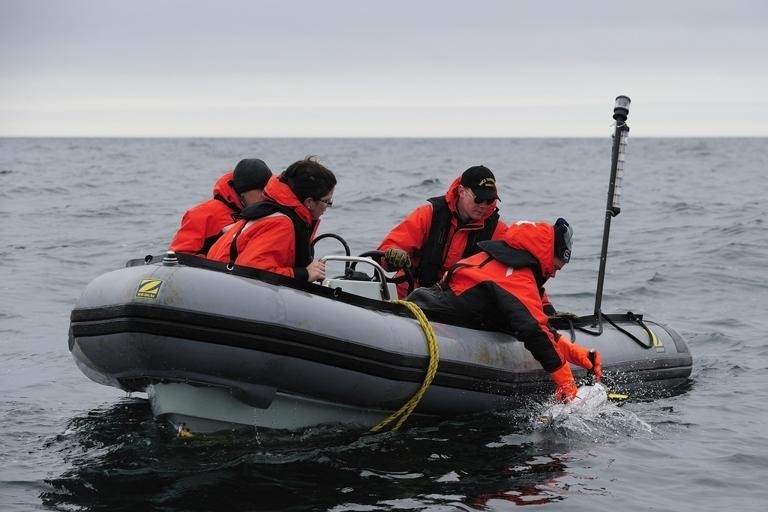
464, 188, 494, 205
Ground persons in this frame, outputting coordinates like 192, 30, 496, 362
401, 216, 602, 406
374, 166, 559, 317
169, 159, 273, 261
202, 152, 338, 284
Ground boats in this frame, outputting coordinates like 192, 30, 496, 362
68, 95, 696, 436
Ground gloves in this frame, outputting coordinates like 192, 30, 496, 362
384, 246, 411, 271
555, 335, 603, 383
550, 359, 578, 405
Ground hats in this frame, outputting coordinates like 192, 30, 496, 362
554, 217, 575, 263
461, 165, 501, 204
233, 159, 273, 195
